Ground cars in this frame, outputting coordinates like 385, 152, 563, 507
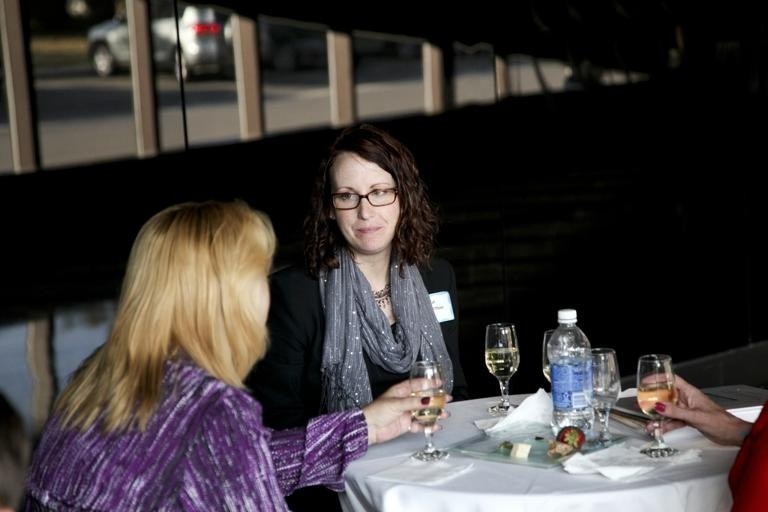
84, 1, 276, 84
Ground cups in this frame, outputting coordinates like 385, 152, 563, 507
541, 329, 556, 382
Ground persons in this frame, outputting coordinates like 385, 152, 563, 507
21, 201, 453, 512
243, 119, 468, 512
633, 370, 768, 511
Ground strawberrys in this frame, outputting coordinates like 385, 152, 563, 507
556, 427, 585, 452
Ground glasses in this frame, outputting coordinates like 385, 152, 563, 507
330, 186, 399, 210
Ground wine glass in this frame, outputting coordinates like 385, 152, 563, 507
639, 353, 674, 453
407, 361, 448, 461
584, 346, 623, 443
484, 321, 520, 414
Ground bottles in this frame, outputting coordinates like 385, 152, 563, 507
550, 307, 593, 440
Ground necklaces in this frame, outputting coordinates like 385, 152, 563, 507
372, 282, 395, 307
